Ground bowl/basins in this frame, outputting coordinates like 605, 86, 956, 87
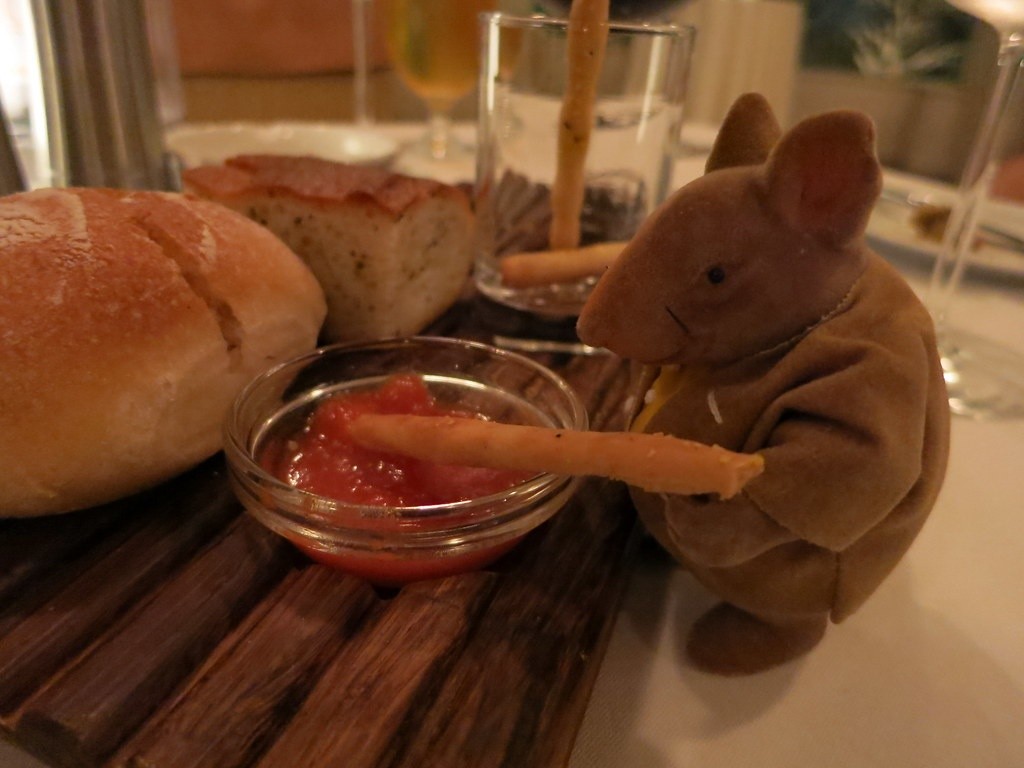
219, 333, 591, 586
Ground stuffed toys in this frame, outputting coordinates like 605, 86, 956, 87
575, 94, 953, 671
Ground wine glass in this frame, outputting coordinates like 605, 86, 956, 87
923, 0, 1024, 422
373, 0, 531, 187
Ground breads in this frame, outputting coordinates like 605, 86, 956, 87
0, 185, 329, 521
182, 152, 473, 341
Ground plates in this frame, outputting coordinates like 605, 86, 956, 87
864, 176, 1023, 277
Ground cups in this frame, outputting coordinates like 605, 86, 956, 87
470, 10, 697, 318
